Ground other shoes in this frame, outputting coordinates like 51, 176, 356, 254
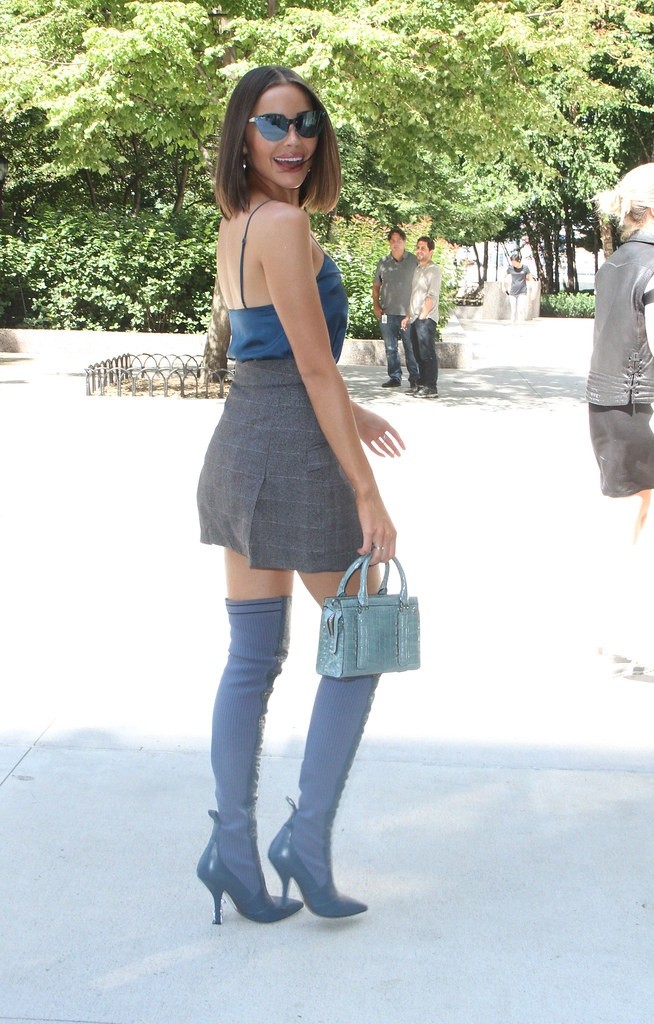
382, 379, 400, 386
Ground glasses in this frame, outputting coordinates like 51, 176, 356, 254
248, 111, 326, 141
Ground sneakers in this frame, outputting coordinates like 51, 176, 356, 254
406, 385, 422, 395
414, 386, 439, 398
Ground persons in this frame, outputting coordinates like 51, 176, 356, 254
587, 162, 654, 682
506, 251, 531, 323
373, 224, 443, 399
197, 63, 409, 925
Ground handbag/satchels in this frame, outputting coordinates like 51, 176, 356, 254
315, 552, 419, 680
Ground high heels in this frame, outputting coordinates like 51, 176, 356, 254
268, 795, 369, 918
197, 810, 303, 925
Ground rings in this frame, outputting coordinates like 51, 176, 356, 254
374, 546, 384, 550
